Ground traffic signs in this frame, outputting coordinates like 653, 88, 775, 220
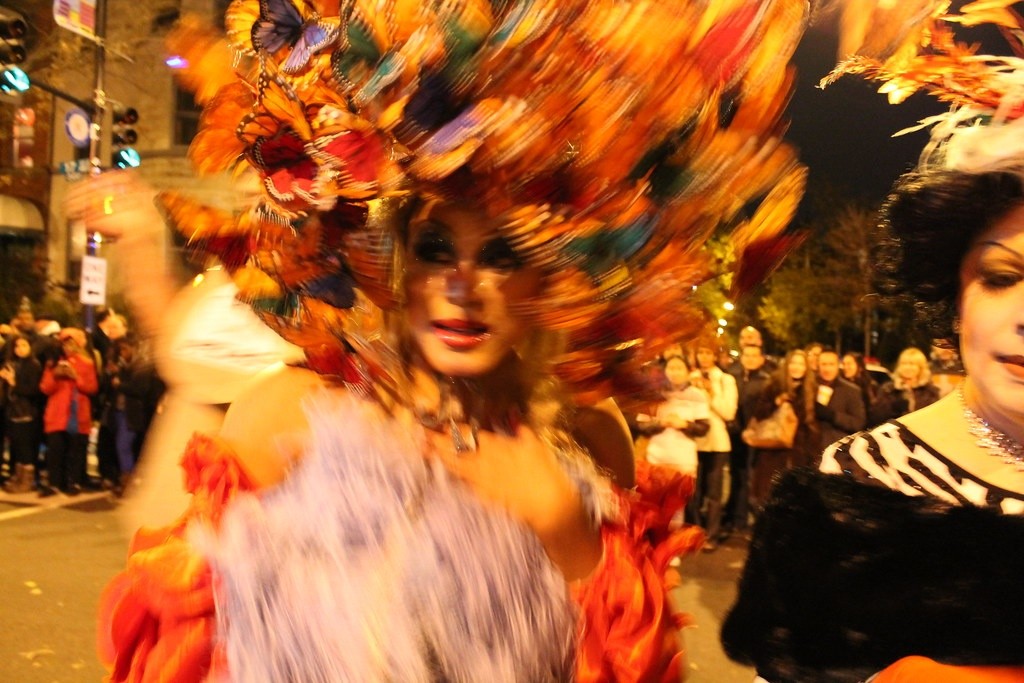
78, 255, 109, 308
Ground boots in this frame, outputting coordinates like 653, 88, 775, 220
5, 463, 34, 492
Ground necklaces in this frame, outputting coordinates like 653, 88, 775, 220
409, 397, 500, 454
958, 384, 1024, 475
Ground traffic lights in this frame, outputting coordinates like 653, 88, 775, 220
0, 11, 33, 95
100, 100, 141, 173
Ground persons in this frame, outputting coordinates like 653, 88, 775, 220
1, 0, 1024, 683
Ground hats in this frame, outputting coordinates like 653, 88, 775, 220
59, 326, 89, 349
0, 323, 14, 335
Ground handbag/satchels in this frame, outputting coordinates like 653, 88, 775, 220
741, 401, 799, 449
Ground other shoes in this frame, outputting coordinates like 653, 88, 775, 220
702, 538, 718, 552
